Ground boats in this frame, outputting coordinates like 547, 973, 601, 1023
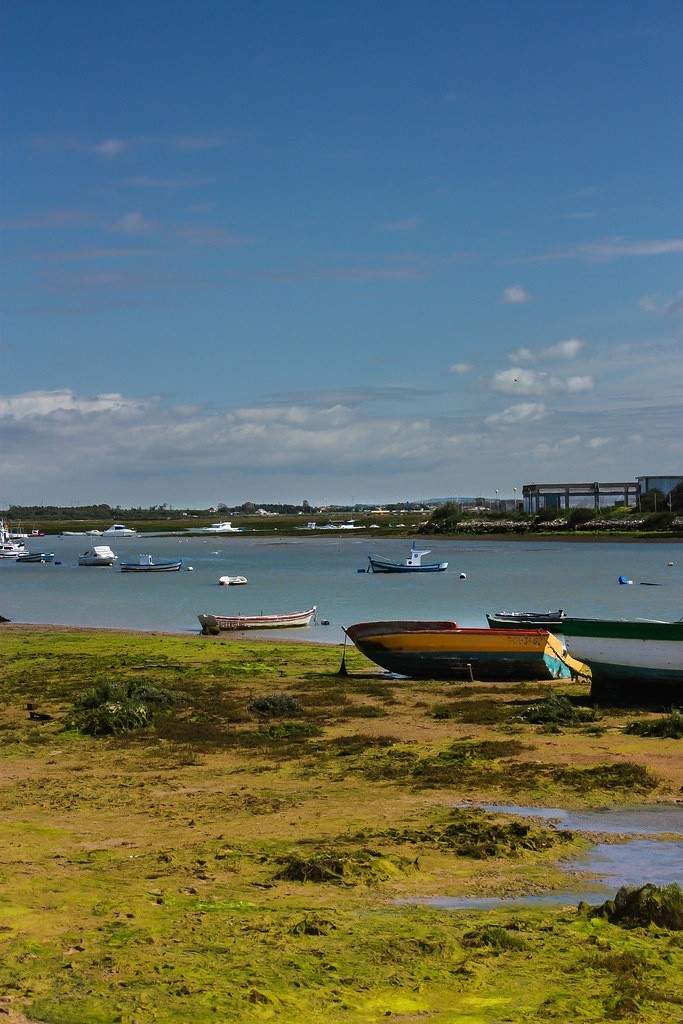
494, 610, 564, 618
0, 545, 30, 558
102, 524, 136, 537
218, 575, 248, 585
78, 546, 118, 566
487, 614, 683, 683
85, 530, 104, 536
341, 620, 593, 680
27, 530, 44, 537
119, 554, 182, 572
62, 531, 84, 536
366, 539, 448, 573
8, 526, 28, 539
18, 552, 54, 562
196, 604, 317, 631
201, 521, 245, 532
